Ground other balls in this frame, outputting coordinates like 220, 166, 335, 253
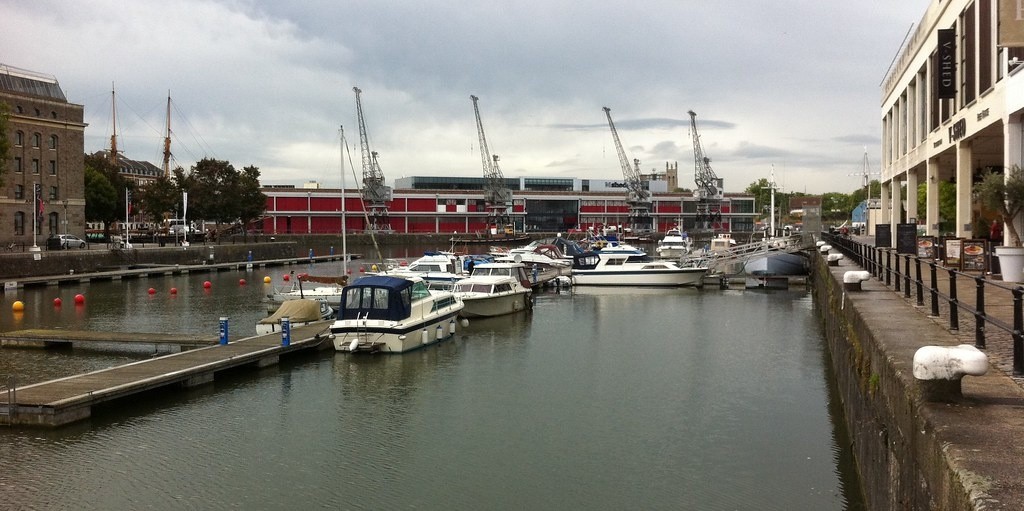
74, 294, 85, 303
53, 298, 62, 305
239, 279, 246, 285
372, 264, 377, 270
148, 288, 155, 294
203, 281, 211, 288
283, 274, 290, 280
264, 276, 271, 283
12, 301, 24, 310
297, 274, 302, 279
360, 267, 364, 272
402, 260, 407, 266
170, 288, 177, 294
303, 273, 308, 276
347, 270, 351, 274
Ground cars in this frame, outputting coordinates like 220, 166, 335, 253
48, 233, 86, 250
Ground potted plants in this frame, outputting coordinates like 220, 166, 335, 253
971, 163, 1024, 282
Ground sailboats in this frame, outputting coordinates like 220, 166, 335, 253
267, 123, 450, 317
83, 80, 247, 242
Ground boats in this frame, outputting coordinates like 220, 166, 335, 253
328, 269, 467, 356
397, 149, 892, 330
255, 298, 332, 336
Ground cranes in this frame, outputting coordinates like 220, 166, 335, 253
601, 107, 656, 239
686, 110, 724, 242
466, 95, 518, 238
351, 86, 393, 230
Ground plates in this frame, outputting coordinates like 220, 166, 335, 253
918, 242, 933, 248
964, 246, 983, 255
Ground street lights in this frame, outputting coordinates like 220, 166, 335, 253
62, 200, 69, 251
